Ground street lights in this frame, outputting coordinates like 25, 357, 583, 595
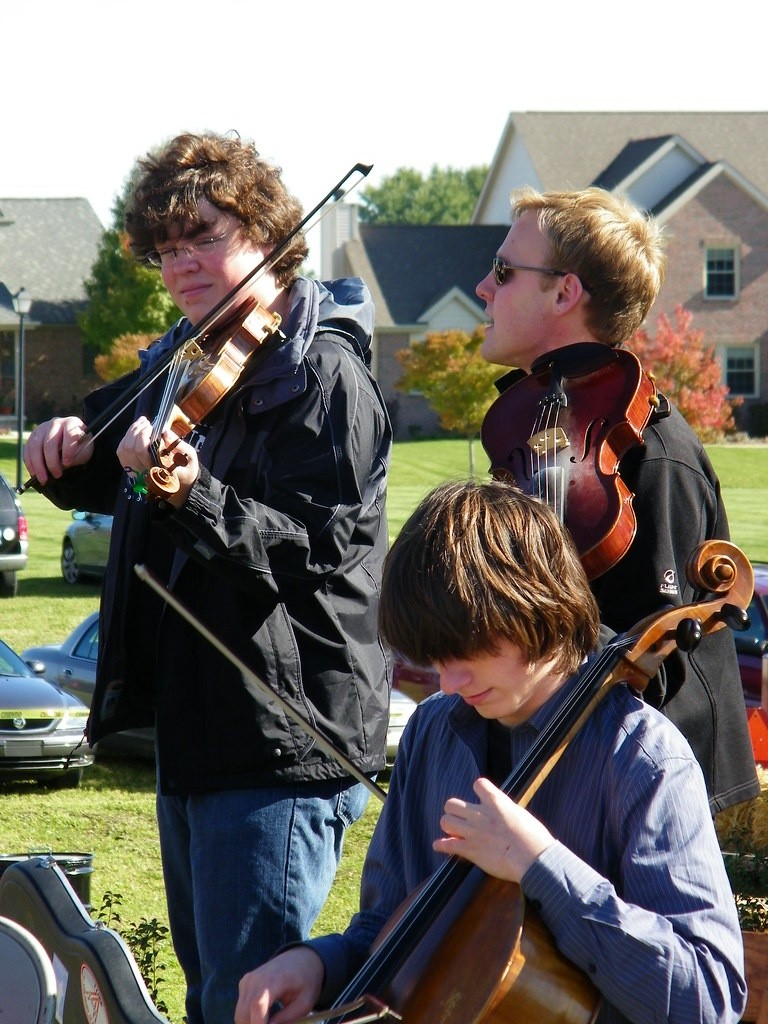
10, 285, 36, 490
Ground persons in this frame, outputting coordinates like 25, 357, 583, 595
22, 129, 394, 1024
233, 189, 760, 1024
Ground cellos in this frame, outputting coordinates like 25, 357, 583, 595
289, 537, 752, 1024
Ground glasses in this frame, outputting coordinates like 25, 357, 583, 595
146, 222, 243, 267
493, 258, 596, 297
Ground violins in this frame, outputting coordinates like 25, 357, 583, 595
137, 298, 285, 497
478, 345, 656, 583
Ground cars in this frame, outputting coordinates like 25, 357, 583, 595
0, 639, 100, 788
730, 556, 768, 772
0, 477, 31, 598
60, 508, 114, 587
19, 608, 418, 780
